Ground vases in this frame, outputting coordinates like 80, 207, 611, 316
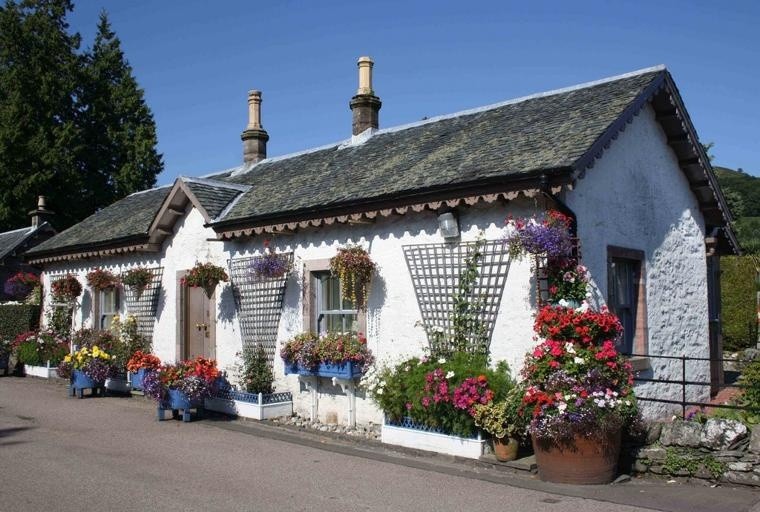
130, 368, 151, 392
156, 389, 205, 422
531, 418, 623, 484
68, 368, 106, 398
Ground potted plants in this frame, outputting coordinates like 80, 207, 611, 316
475, 386, 529, 462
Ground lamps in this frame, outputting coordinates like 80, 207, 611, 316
438, 212, 459, 238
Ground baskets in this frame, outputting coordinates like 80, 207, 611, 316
130, 285, 145, 302
199, 281, 218, 300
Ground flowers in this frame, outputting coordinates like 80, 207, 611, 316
126, 352, 161, 374
145, 361, 211, 405
59, 345, 118, 384
519, 306, 641, 425
